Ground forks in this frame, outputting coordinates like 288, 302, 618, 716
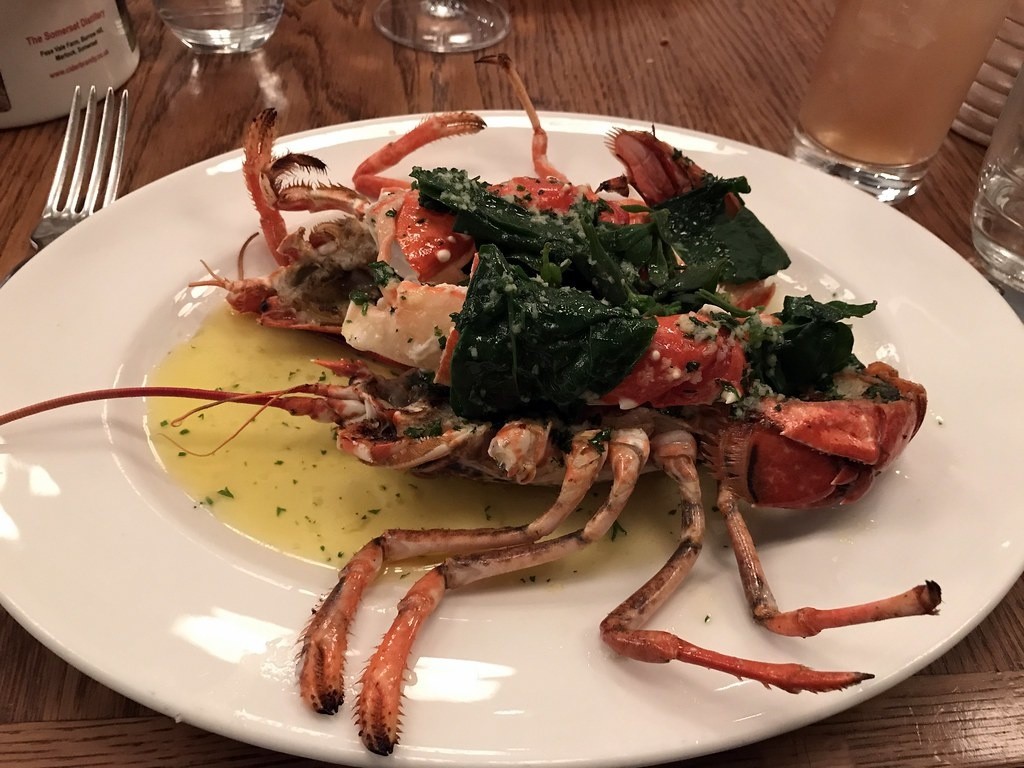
0, 86, 129, 287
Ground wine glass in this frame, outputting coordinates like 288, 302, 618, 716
375, 0, 512, 53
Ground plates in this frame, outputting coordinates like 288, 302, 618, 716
0, 109, 1024, 768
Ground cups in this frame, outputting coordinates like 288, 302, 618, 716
789, 0, 1016, 205
154, 0, 284, 54
972, 66, 1024, 292
